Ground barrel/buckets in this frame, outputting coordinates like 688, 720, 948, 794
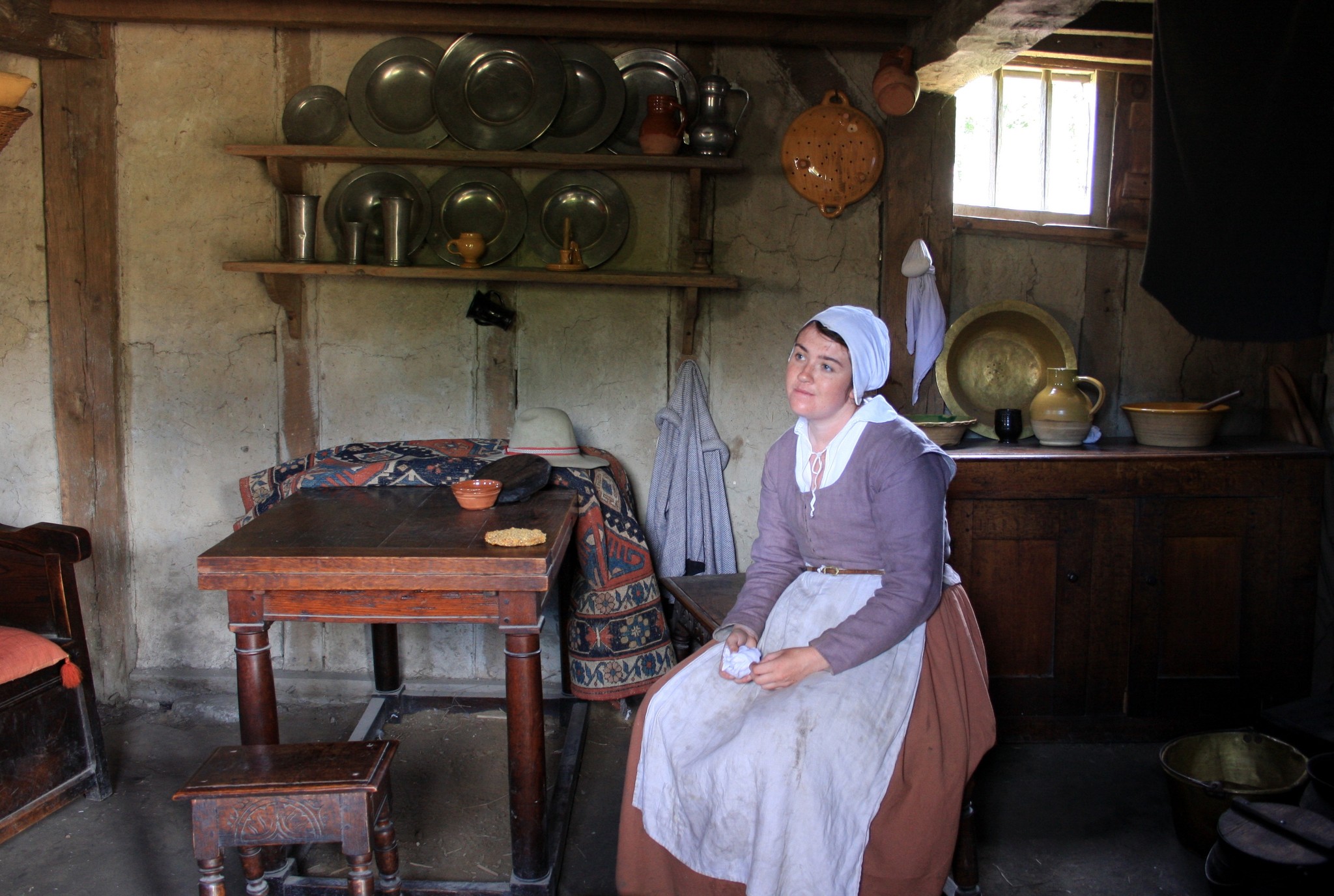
1158, 728, 1311, 855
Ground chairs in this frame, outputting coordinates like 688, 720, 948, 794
1, 522, 112, 843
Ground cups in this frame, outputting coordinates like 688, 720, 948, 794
994, 409, 1022, 444
283, 194, 321, 264
340, 222, 364, 265
379, 197, 414, 267
466, 290, 516, 331
447, 233, 487, 268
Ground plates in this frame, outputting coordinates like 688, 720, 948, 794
282, 86, 349, 144
434, 33, 566, 150
324, 164, 432, 262
427, 167, 528, 266
345, 37, 448, 149
604, 49, 701, 156
532, 42, 626, 152
524, 169, 629, 268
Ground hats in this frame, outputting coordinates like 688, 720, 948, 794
474, 407, 610, 469
788, 305, 890, 405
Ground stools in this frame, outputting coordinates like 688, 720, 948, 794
172, 739, 403, 896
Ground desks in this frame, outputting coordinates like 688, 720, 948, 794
195, 487, 587, 896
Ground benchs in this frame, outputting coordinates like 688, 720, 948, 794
660, 571, 981, 896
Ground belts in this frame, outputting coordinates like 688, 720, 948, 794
806, 567, 886, 575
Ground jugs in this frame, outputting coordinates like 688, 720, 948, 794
640, 95, 688, 155
872, 46, 920, 115
1030, 368, 1105, 446
670, 69, 749, 157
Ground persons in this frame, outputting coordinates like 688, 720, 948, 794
616, 304, 995, 896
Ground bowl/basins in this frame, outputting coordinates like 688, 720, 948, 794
936, 300, 1078, 440
900, 414, 977, 447
1119, 403, 1234, 447
451, 479, 502, 509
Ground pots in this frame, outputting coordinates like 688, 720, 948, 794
1216, 796, 1334, 884
781, 90, 883, 218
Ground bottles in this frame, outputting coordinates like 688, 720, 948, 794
1299, 752, 1334, 821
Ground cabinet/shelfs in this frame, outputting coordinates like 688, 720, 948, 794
929, 438, 1328, 738
223, 143, 741, 292
